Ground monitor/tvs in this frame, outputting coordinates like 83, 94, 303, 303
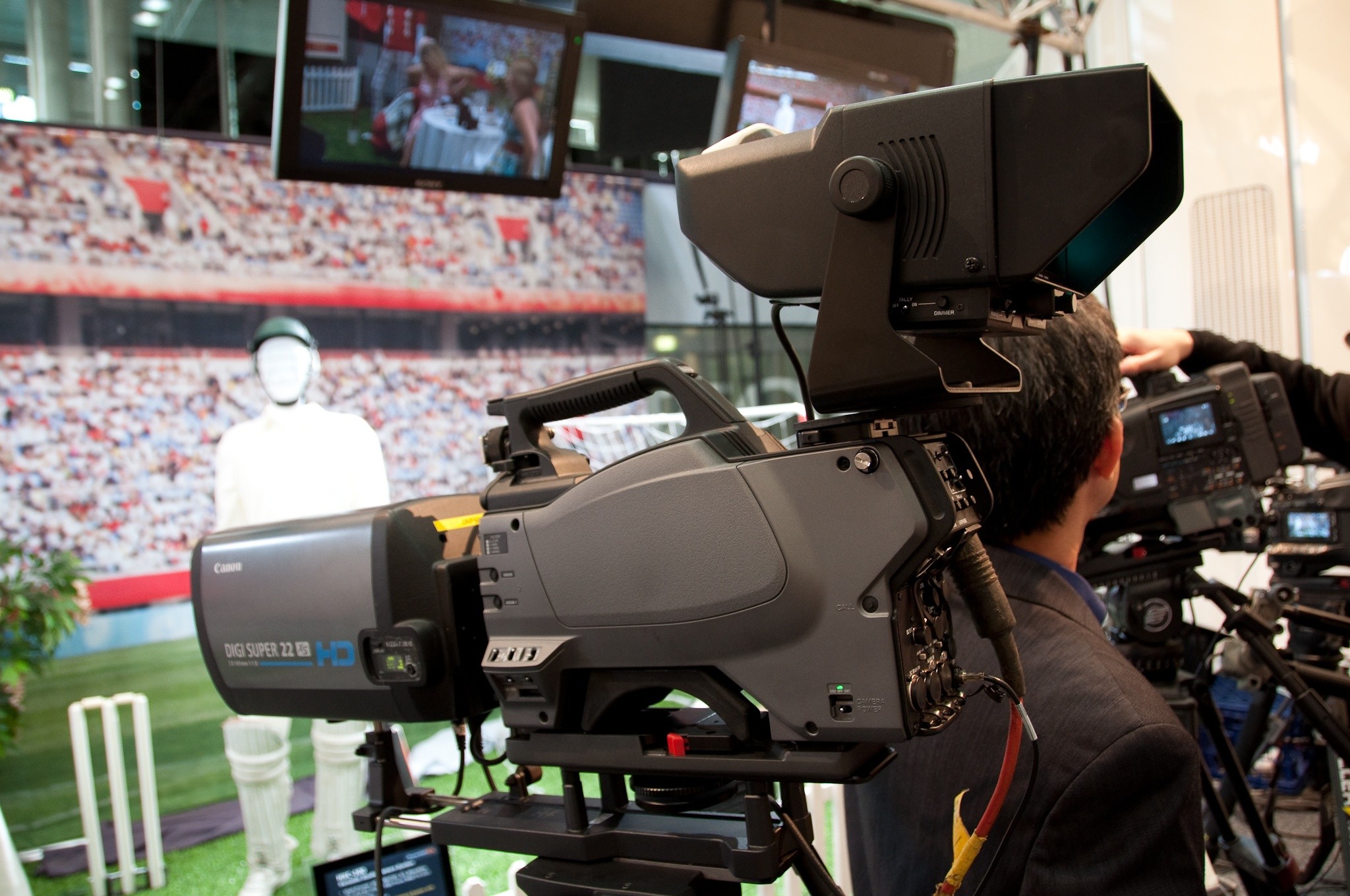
272, 0, 586, 200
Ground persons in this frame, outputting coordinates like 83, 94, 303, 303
1113, 327, 1350, 472
845, 290, 1210, 896
213, 317, 388, 896
369, 40, 547, 183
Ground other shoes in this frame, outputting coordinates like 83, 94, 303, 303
1249, 748, 1327, 809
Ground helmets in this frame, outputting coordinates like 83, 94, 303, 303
248, 317, 313, 352
